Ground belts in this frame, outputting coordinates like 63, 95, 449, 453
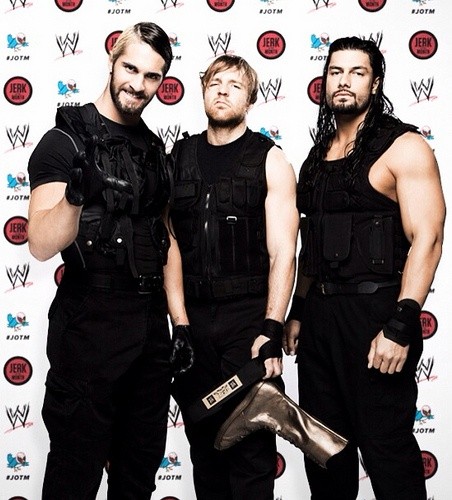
311, 280, 400, 296
83, 273, 163, 295
183, 273, 267, 300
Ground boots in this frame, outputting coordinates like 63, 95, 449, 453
214, 377, 349, 472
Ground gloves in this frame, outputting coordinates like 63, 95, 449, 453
169, 324, 203, 386
65, 135, 133, 206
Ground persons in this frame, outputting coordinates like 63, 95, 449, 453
25, 21, 170, 499
280, 36, 447, 500
159, 55, 299, 500
